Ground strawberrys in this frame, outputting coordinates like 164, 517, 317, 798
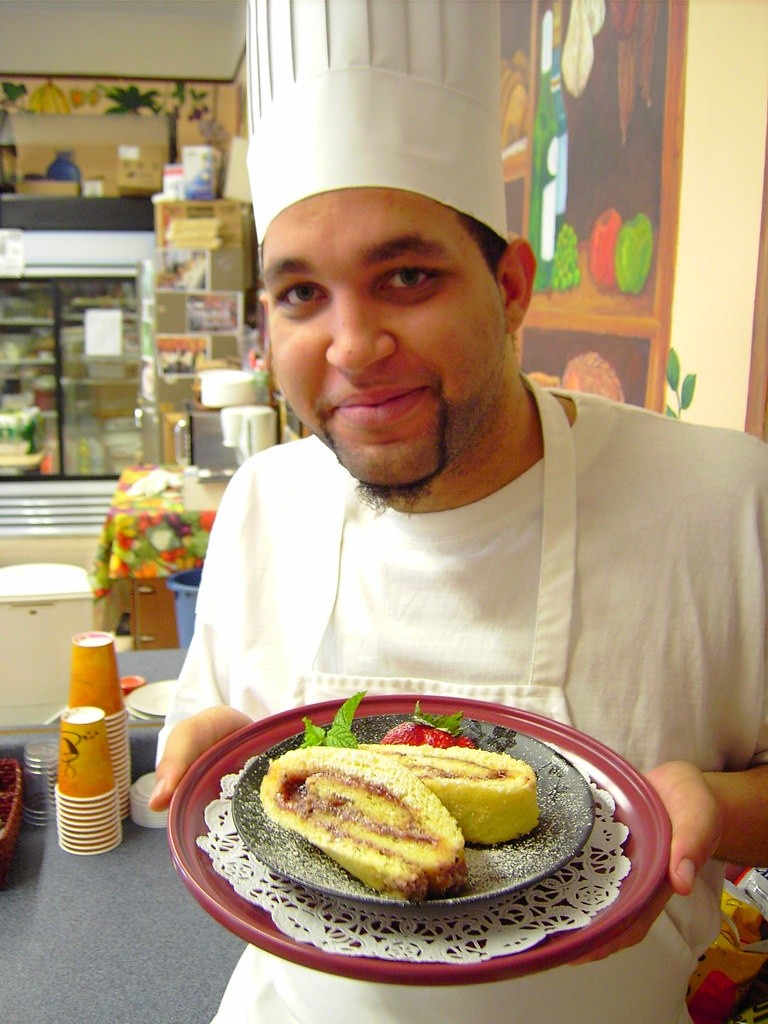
380, 699, 476, 750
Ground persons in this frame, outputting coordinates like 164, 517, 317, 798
147, 0, 767, 1024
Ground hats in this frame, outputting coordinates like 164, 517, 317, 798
242, 0, 508, 256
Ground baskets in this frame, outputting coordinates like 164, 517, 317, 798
0, 758, 22, 883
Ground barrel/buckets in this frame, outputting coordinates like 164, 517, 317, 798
165, 568, 202, 649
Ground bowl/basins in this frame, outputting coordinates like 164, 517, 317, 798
119, 676, 146, 695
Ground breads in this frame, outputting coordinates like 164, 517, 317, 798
258, 745, 472, 903
356, 742, 541, 846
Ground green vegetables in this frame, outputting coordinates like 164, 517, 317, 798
298, 690, 368, 748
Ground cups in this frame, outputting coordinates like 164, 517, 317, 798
22, 631, 132, 857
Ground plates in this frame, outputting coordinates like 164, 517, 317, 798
168, 694, 672, 987
230, 713, 596, 908
129, 772, 169, 829
122, 680, 177, 721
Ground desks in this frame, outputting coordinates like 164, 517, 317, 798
0, 727, 768, 1024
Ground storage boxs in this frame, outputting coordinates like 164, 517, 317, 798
148, 195, 254, 277
